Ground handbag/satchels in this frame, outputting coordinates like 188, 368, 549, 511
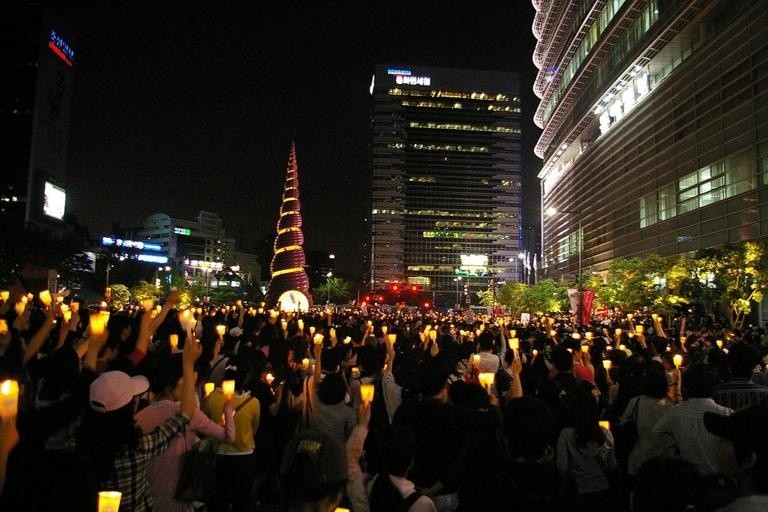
173, 448, 217, 504
494, 369, 512, 391
615, 415, 639, 457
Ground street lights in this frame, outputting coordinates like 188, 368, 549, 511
326, 271, 332, 302
545, 207, 583, 290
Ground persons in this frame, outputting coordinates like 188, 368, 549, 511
1, 285, 767, 512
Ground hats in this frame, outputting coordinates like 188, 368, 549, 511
89, 370, 150, 412
703, 405, 767, 448
280, 429, 350, 483
